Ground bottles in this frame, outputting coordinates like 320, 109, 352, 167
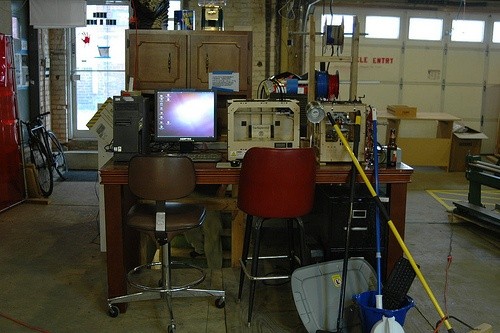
387, 130, 397, 168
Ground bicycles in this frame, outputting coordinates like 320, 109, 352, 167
15, 112, 68, 198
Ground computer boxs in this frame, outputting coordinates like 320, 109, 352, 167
112, 96, 151, 163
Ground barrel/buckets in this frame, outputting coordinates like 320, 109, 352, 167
353, 292, 415, 333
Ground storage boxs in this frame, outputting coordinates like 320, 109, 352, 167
315, 185, 389, 271
388, 104, 417, 116
449, 125, 488, 171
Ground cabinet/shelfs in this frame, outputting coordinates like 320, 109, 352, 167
126, 30, 253, 129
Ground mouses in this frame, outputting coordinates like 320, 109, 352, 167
231, 160, 240, 167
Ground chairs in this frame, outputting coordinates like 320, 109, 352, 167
237, 147, 318, 326
104, 156, 225, 333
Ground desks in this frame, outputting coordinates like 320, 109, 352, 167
376, 111, 461, 170
100, 155, 414, 314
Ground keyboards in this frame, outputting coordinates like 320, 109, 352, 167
158, 152, 221, 162
381, 256, 420, 310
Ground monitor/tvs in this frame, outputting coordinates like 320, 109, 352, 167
154, 88, 217, 154
